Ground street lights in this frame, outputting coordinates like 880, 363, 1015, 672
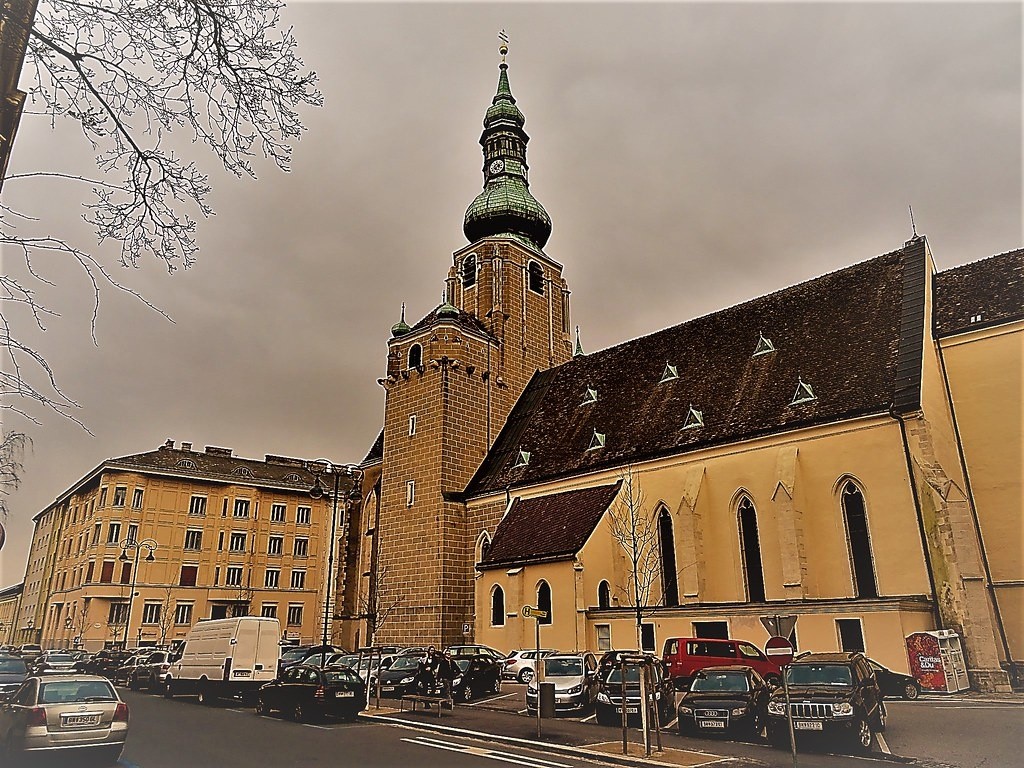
118, 538, 158, 649
310, 458, 365, 668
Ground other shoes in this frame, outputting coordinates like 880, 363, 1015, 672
425, 703, 431, 709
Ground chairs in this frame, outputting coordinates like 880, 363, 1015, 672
76, 686, 90, 697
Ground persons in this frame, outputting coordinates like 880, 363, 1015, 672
436, 649, 461, 710
416, 646, 439, 709
85, 656, 108, 676
9, 652, 21, 658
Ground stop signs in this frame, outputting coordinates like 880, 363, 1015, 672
765, 636, 794, 667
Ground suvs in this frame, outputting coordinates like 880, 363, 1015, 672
502, 648, 560, 684
526, 650, 598, 716
766, 650, 883, 753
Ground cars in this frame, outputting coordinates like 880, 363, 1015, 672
0, 641, 508, 768
866, 657, 921, 701
0, 673, 131, 768
256, 662, 367, 725
676, 664, 770, 740
597, 649, 672, 727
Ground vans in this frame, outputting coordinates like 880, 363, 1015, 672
162, 615, 283, 706
661, 637, 783, 693
